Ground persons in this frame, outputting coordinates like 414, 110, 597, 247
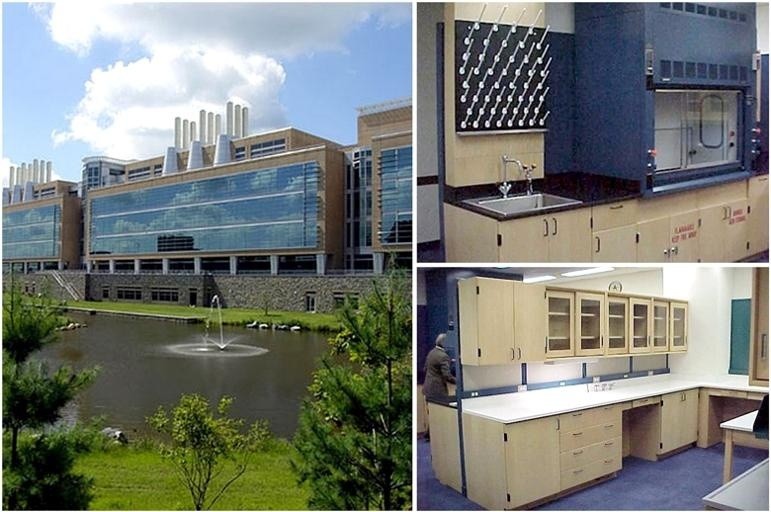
422, 334, 456, 442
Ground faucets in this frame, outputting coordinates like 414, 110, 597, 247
499, 154, 524, 199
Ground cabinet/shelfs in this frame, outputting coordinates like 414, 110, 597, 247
458, 277, 690, 366
444, 172, 769, 263
559, 403, 624, 494
630, 387, 699, 463
462, 414, 559, 511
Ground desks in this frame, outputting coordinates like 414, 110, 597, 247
719, 408, 769, 485
702, 455, 769, 511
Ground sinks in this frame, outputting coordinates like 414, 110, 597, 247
477, 190, 583, 216
